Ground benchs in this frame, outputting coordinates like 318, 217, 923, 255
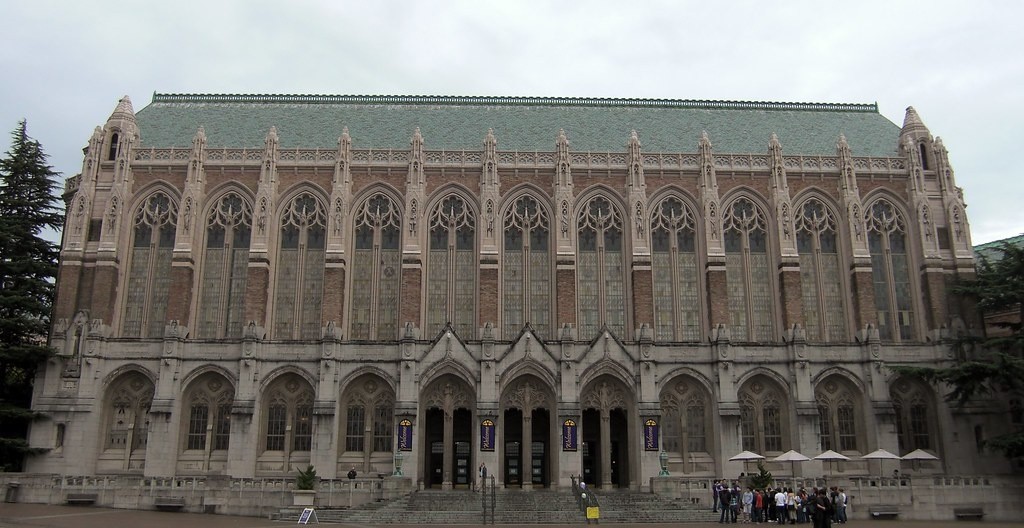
64, 493, 98, 507
953, 507, 988, 521
869, 505, 903, 520
151, 497, 185, 512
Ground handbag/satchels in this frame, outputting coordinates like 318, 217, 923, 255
794, 502, 797, 509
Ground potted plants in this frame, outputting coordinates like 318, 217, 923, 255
752, 471, 772, 493
291, 464, 317, 505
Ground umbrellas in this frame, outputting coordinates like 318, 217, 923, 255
811, 450, 851, 475
729, 450, 766, 473
859, 448, 902, 476
774, 450, 811, 476
901, 449, 941, 468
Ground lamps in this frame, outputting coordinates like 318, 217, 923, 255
393, 450, 403, 476
659, 450, 670, 475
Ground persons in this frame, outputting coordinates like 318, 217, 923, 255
712, 479, 848, 528
347, 467, 357, 489
892, 469, 899, 479
477, 462, 487, 492
